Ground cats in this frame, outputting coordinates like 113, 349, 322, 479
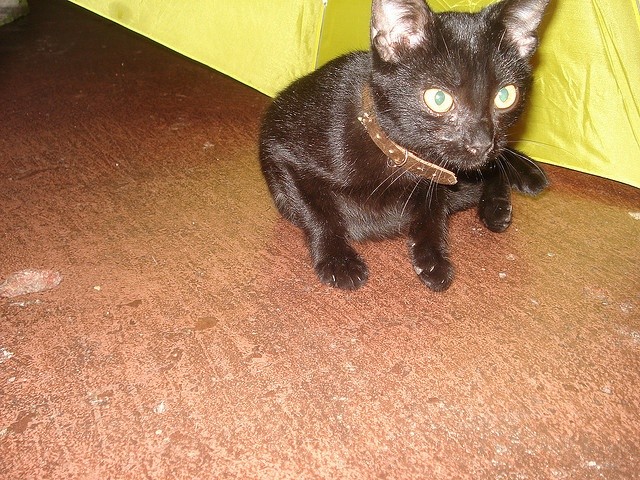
257, 0, 550, 291
